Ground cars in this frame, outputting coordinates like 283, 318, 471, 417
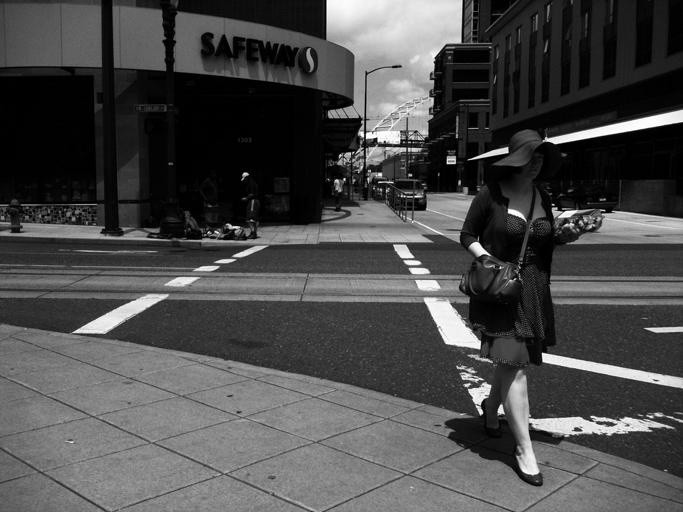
371, 176, 428, 211
540, 180, 620, 213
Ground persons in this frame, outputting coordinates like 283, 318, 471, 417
240, 172, 260, 239
325, 175, 357, 194
199, 169, 220, 231
460, 129, 583, 485
333, 175, 344, 211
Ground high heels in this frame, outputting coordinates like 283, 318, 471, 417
481, 398, 503, 438
512, 448, 543, 486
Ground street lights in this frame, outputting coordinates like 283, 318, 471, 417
363, 64, 402, 201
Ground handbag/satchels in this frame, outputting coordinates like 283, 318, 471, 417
459, 254, 522, 304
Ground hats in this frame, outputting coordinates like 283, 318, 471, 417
241, 172, 250, 182
491, 129, 559, 167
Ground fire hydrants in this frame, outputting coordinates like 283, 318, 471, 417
6, 198, 23, 234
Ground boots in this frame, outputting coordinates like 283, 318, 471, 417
247, 231, 257, 239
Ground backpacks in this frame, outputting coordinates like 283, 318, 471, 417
207, 223, 246, 240
